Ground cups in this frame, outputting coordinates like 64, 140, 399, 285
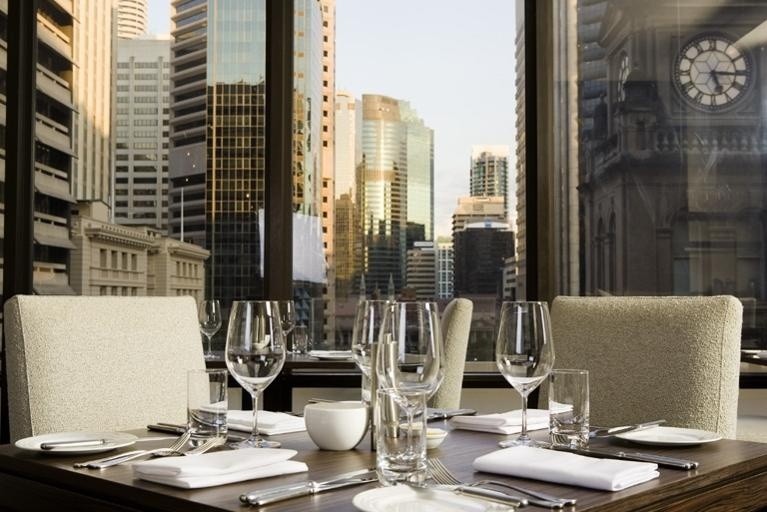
290, 324, 309, 355
350, 295, 445, 485
186, 365, 227, 449
547, 366, 590, 449
302, 402, 370, 451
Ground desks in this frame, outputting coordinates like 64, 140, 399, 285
1, 418, 766, 512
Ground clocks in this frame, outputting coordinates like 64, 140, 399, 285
668, 28, 759, 115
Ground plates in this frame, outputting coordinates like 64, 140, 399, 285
608, 425, 723, 448
15, 430, 137, 457
311, 353, 353, 361
351, 483, 514, 511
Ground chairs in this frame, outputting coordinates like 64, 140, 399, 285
1, 294, 210, 444
421, 295, 475, 412
534, 292, 744, 439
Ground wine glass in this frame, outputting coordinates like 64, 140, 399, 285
227, 299, 282, 448
279, 300, 296, 357
494, 299, 554, 450
197, 299, 222, 358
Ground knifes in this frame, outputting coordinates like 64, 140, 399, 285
147, 421, 245, 441
397, 477, 528, 507
238, 468, 378, 505
425, 408, 475, 421
39, 436, 177, 449
575, 446, 697, 471
593, 418, 663, 435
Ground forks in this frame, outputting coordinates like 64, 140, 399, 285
425, 458, 576, 508
88, 439, 217, 470
73, 431, 190, 469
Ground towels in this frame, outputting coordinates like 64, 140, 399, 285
448, 408, 549, 436
130, 445, 310, 491
226, 409, 307, 436
472, 443, 661, 492
